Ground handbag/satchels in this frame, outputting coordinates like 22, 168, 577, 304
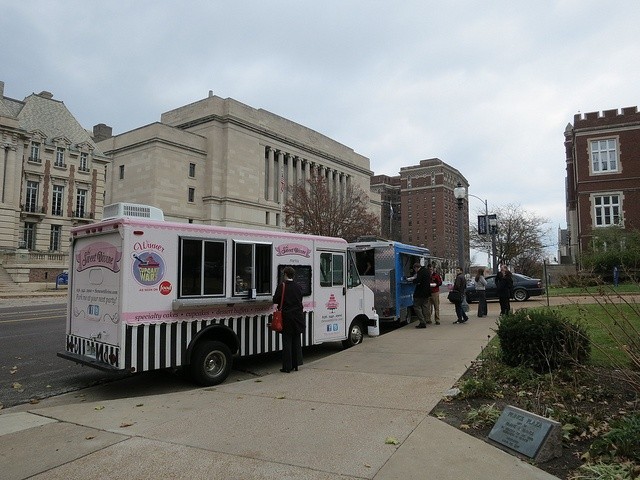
272, 282, 285, 332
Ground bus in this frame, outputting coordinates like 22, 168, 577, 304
348, 236, 431, 325
55, 203, 380, 387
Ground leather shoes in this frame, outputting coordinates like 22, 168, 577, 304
416, 324, 426, 328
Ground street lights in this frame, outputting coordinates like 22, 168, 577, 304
454, 180, 491, 273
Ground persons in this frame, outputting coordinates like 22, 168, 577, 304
271, 265, 303, 374
495, 265, 515, 318
474, 267, 488, 319
406, 262, 429, 329
447, 266, 469, 324
427, 264, 442, 328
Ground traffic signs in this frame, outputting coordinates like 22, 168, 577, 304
478, 215, 497, 234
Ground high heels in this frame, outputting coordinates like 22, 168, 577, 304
292, 366, 298, 371
280, 368, 290, 373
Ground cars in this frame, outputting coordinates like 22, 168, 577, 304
464, 271, 544, 303
